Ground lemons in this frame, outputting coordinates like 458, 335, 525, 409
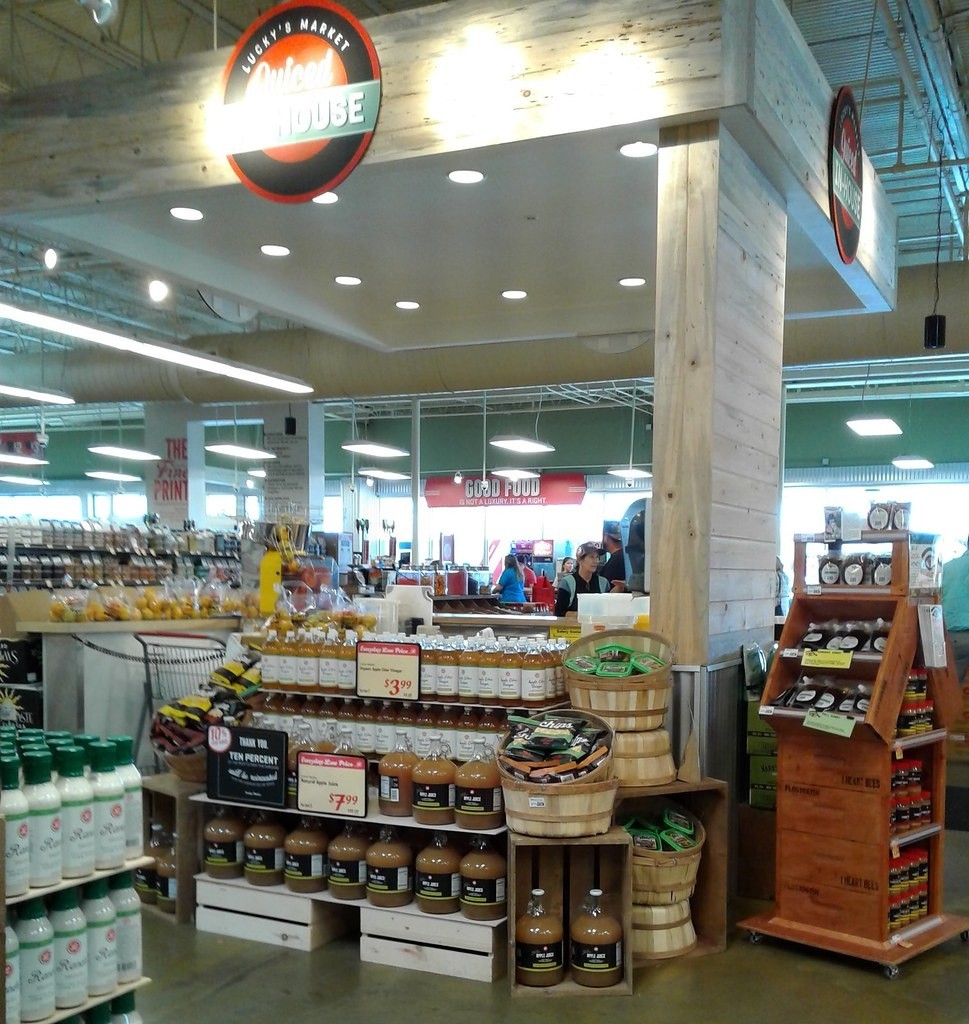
277, 525, 298, 572
269, 610, 377, 637
47, 587, 262, 622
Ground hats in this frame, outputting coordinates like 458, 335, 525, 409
603, 521, 622, 541
576, 542, 606, 561
517, 555, 526, 565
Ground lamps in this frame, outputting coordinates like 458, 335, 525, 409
606, 380, 653, 481
0, 300, 554, 485
844, 363, 903, 437
891, 382, 935, 469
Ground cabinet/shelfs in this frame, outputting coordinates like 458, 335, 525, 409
735, 587, 969, 978
0, 539, 571, 1024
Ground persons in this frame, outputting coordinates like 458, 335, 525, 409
599, 525, 626, 593
553, 543, 626, 618
942, 536, 969, 681
552, 557, 574, 591
492, 554, 527, 602
774, 557, 790, 641
517, 555, 536, 602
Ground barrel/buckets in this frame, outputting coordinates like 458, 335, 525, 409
606, 728, 676, 788
561, 629, 673, 682
566, 678, 671, 732
497, 708, 615, 784
626, 807, 706, 959
501, 776, 618, 838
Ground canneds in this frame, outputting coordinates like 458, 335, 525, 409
893, 666, 934, 738
890, 758, 931, 834
888, 848, 928, 932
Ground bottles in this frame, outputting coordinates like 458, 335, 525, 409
570, 889, 623, 986
352, 564, 492, 596
134, 823, 167, 904
156, 832, 177, 914
203, 626, 568, 920
0, 722, 144, 1024
515, 889, 564, 987
